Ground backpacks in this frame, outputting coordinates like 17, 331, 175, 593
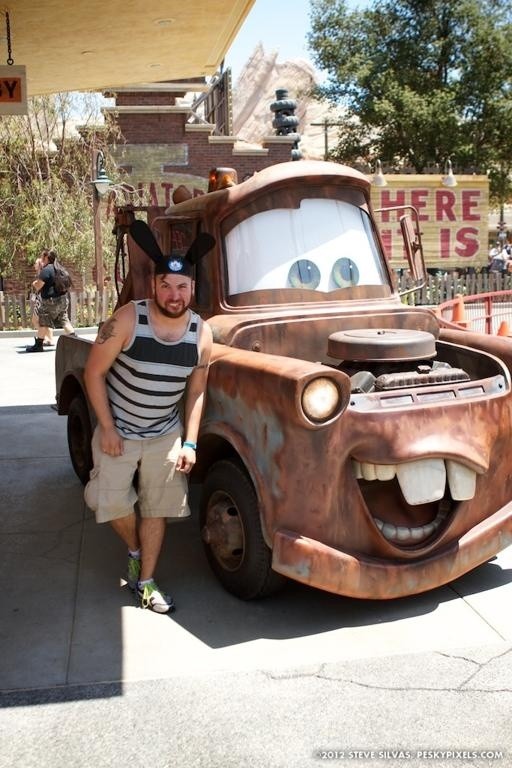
51, 259, 71, 295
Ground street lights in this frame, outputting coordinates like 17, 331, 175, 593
89, 147, 112, 323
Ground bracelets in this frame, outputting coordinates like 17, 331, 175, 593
182, 441, 198, 450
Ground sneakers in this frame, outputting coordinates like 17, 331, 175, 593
135, 580, 176, 613
124, 553, 140, 592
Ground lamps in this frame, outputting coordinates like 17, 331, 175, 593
371, 155, 463, 192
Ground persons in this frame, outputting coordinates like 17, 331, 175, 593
83, 254, 213, 614
394, 239, 512, 306
26, 247, 78, 352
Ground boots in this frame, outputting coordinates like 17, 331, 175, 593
25, 337, 43, 352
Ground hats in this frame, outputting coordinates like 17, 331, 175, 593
153, 252, 195, 276
